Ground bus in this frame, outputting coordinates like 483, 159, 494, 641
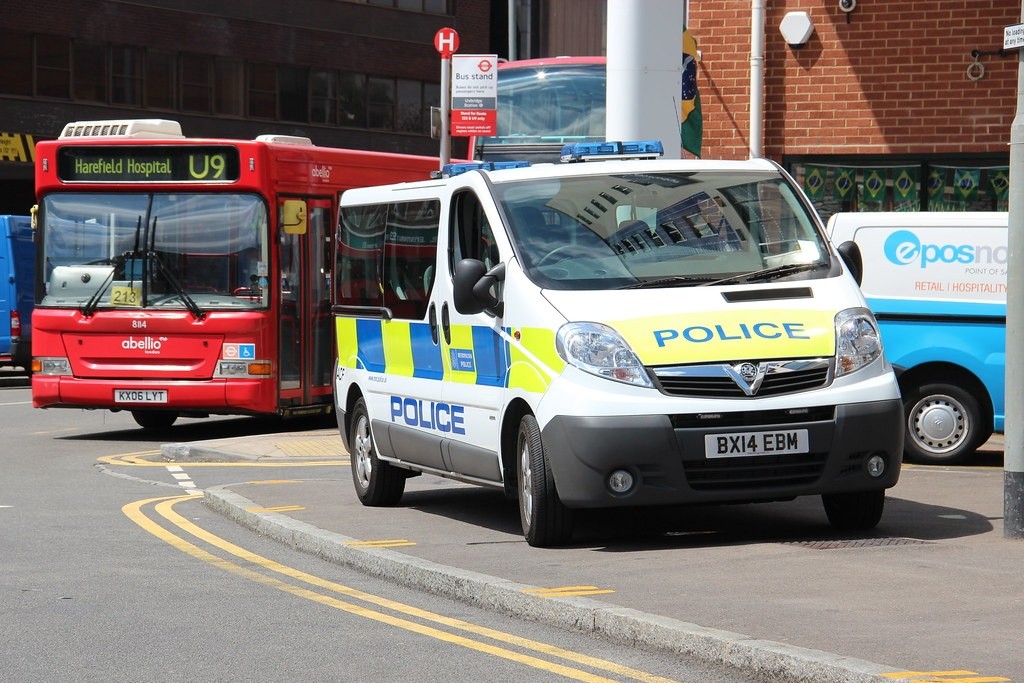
425, 55, 667, 163
33, 118, 489, 431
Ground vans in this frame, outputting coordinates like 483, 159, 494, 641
0, 213, 34, 382
821, 208, 1011, 464
334, 138, 905, 548
